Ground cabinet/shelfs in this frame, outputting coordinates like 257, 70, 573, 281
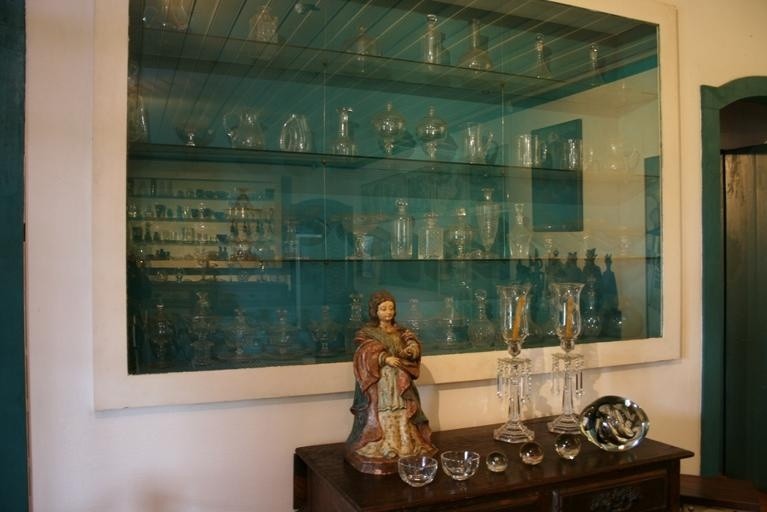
293, 413, 696, 512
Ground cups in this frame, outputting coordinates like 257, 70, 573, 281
223, 107, 265, 149
606, 138, 638, 176
328, 213, 389, 260
440, 450, 481, 481
513, 134, 548, 167
461, 124, 494, 164
397, 455, 438, 489
562, 138, 597, 172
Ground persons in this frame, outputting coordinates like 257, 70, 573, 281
342, 291, 439, 476
